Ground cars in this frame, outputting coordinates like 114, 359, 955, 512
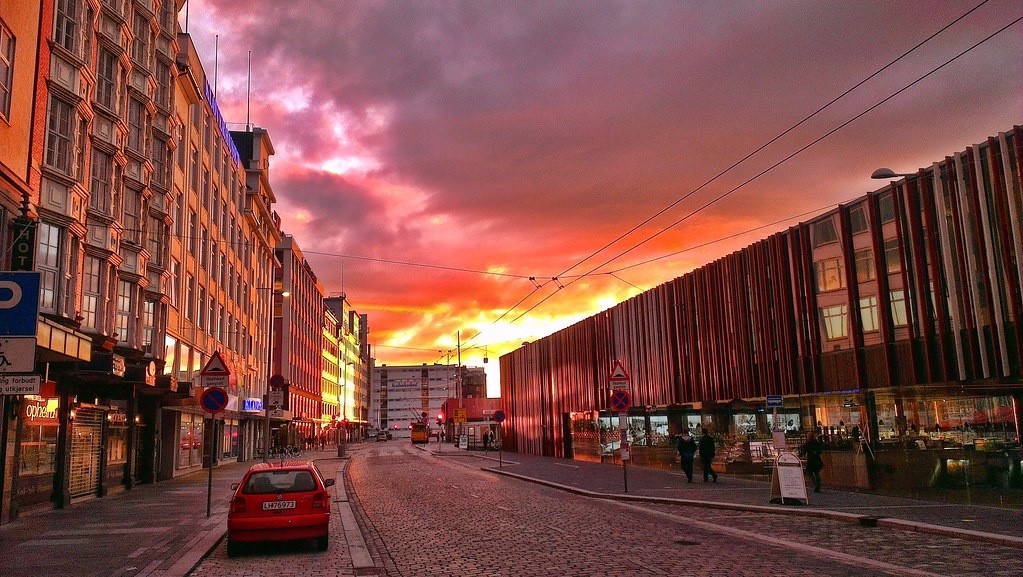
377, 432, 387, 441
227, 460, 336, 554
369, 432, 377, 438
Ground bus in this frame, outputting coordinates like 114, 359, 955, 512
410, 422, 430, 443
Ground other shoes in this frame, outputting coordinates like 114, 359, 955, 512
813, 489, 820, 493
713, 476, 718, 482
704, 477, 709, 482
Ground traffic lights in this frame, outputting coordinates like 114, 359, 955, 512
439, 417, 442, 425
436, 418, 439, 425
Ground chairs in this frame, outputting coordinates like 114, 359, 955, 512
253, 477, 273, 492
294, 473, 313, 489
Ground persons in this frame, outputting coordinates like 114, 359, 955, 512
801, 431, 823, 493
677, 430, 697, 483
698, 428, 718, 483
300, 433, 326, 450
483, 432, 493, 452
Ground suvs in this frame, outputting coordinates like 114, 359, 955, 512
380, 431, 393, 440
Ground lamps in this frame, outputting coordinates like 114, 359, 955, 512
522, 342, 529, 345
870, 168, 916, 179
272, 291, 290, 297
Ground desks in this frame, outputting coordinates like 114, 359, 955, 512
937, 449, 988, 484
